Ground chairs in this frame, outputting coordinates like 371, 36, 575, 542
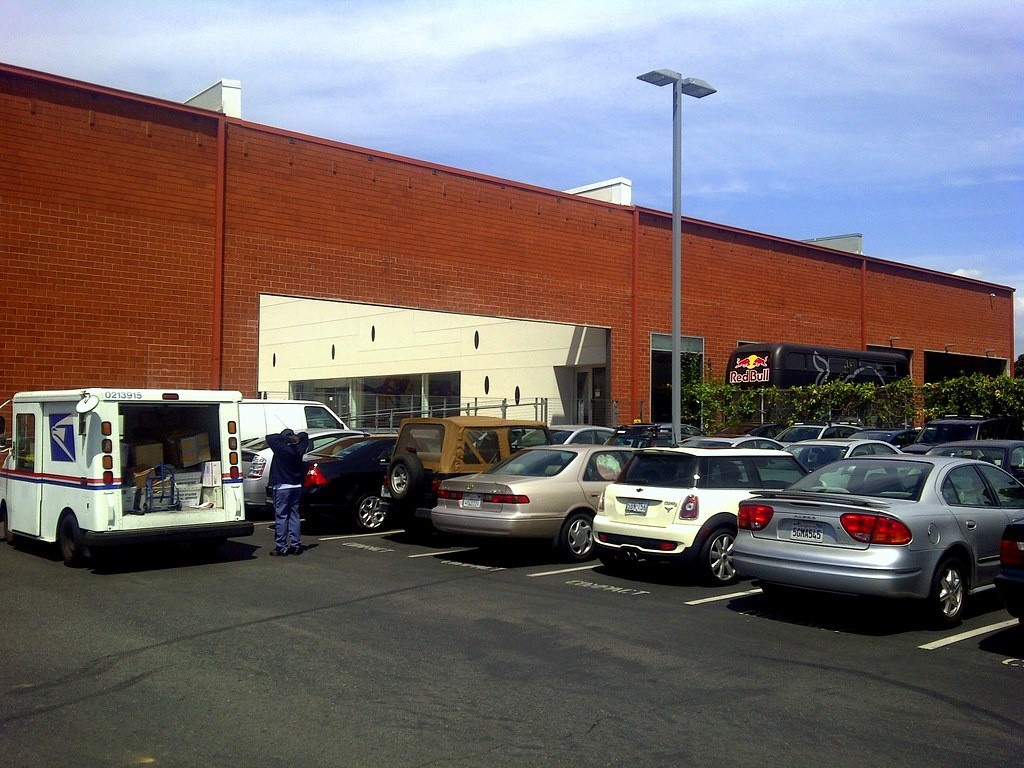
860, 474, 900, 496
719, 462, 743, 486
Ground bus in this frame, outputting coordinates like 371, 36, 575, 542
724, 340, 910, 427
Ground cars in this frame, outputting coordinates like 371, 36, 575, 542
512, 411, 1023, 483
588, 442, 825, 588
731, 456, 1023, 627
240, 428, 378, 509
264, 432, 444, 532
429, 443, 661, 564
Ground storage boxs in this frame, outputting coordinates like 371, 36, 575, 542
118, 432, 224, 515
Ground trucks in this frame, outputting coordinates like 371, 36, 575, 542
0, 383, 256, 570
239, 396, 353, 450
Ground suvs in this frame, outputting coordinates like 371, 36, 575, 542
382, 415, 564, 522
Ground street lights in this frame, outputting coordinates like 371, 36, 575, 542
637, 69, 720, 449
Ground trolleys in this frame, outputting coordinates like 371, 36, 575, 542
141, 463, 182, 514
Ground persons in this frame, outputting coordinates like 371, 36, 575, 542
265, 429, 309, 556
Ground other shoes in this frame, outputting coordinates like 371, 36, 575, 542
288, 547, 302, 555
270, 547, 288, 556
